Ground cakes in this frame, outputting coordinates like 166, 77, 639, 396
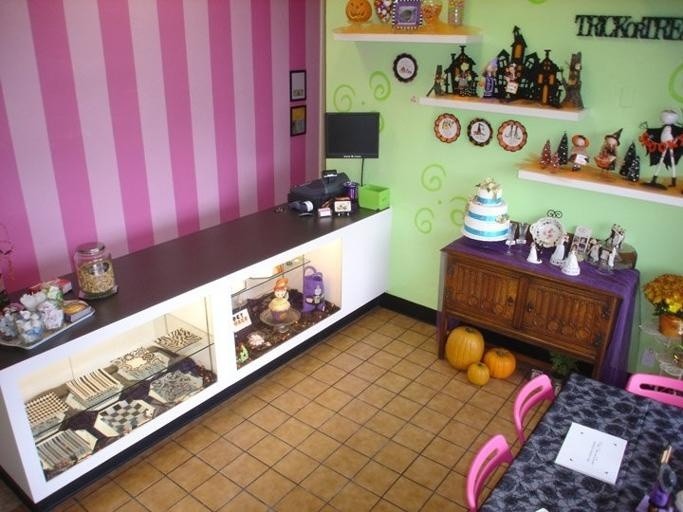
463, 178, 511, 240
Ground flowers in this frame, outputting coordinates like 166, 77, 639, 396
644, 273, 683, 316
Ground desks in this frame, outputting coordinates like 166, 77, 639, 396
476, 373, 683, 512
641, 321, 683, 396
0, 197, 391, 511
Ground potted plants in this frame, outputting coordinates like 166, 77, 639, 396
548, 351, 579, 384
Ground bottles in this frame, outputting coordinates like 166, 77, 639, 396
74, 241, 116, 295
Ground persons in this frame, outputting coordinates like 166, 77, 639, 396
594, 126, 623, 177
568, 135, 590, 170
639, 109, 683, 189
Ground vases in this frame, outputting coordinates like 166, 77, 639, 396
658, 311, 683, 337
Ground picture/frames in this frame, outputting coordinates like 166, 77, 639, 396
290, 105, 307, 136
392, 1, 422, 30
289, 70, 307, 102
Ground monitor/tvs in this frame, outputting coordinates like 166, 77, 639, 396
324, 112, 380, 159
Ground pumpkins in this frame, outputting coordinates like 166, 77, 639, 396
444, 326, 485, 372
484, 348, 517, 379
467, 362, 490, 384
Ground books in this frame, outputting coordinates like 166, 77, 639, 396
63, 299, 92, 322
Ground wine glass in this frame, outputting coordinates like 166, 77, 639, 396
505, 221, 529, 256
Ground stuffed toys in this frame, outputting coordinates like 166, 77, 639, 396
302, 272, 326, 312
268, 277, 292, 321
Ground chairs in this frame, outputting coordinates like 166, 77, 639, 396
466, 435, 514, 511
514, 375, 555, 445
625, 374, 683, 410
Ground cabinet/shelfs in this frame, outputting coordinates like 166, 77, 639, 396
437, 234, 639, 381
332, 25, 683, 207
